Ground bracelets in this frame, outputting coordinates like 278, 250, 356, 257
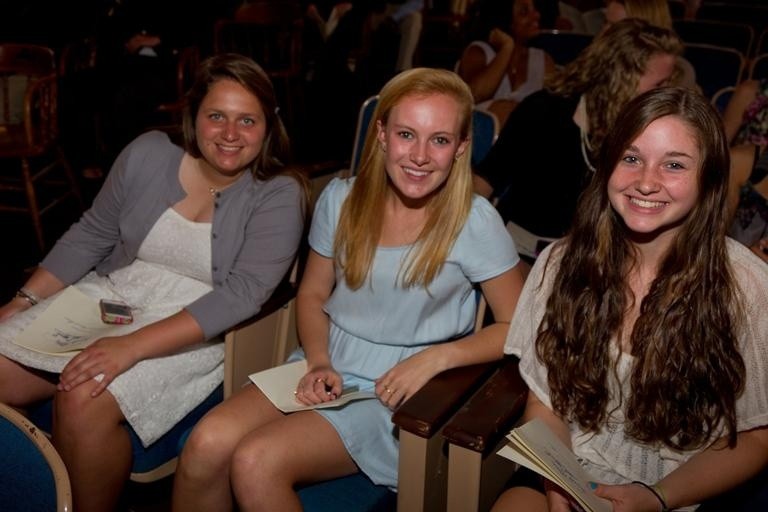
653, 483, 665, 512
14, 287, 37, 306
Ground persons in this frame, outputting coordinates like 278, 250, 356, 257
307, 2, 767, 262
486, 83, 767, 512
170, 66, 525, 512
59, 2, 192, 207
0, 53, 312, 512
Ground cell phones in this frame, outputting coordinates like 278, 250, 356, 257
100, 299, 133, 324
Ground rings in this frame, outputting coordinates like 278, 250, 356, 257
384, 385, 393, 394
294, 391, 297, 394
315, 379, 325, 385
